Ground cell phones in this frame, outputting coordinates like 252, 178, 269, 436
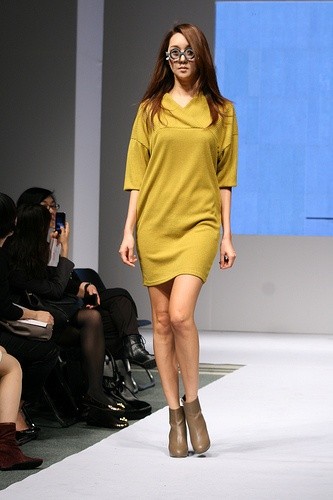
85, 293, 97, 306
55, 212, 65, 234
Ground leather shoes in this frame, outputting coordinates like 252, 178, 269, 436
84, 413, 129, 429
14, 425, 40, 435
83, 393, 125, 412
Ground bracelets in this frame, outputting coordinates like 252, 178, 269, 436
84, 282, 93, 294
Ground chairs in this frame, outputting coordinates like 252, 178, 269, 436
15, 269, 155, 446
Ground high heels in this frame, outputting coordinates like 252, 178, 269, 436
168, 405, 188, 458
121, 334, 157, 372
181, 393, 211, 453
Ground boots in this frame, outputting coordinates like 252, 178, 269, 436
0, 422, 43, 470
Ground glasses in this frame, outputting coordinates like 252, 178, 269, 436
43, 204, 60, 211
166, 48, 196, 60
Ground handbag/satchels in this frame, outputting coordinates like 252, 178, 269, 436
1, 305, 53, 341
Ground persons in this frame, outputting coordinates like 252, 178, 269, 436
118, 23, 239, 459
0, 187, 158, 470
